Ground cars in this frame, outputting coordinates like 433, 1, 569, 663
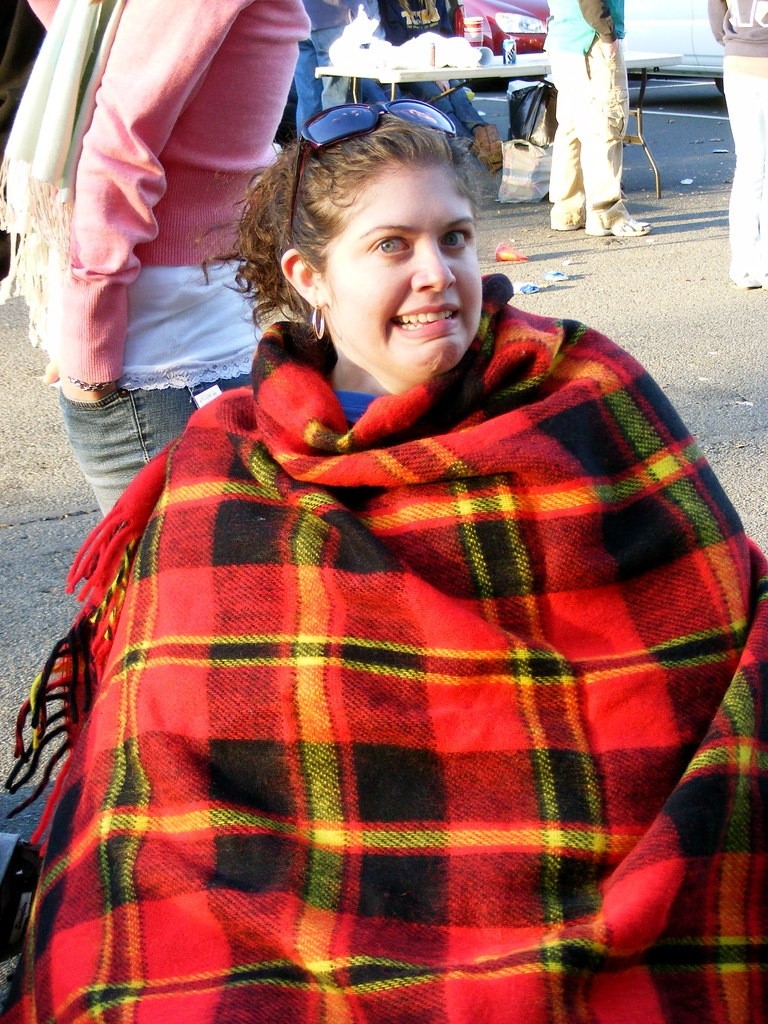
449, 0, 550, 56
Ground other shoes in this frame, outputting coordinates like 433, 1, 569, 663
728, 266, 766, 289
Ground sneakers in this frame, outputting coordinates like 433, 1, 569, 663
549, 212, 585, 231
585, 213, 652, 239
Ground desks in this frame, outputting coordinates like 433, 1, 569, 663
314, 50, 682, 200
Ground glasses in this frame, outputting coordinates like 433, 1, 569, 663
283, 98, 458, 238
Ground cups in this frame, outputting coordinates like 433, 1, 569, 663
463, 17, 494, 67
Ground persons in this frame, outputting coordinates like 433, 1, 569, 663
0, 0, 311, 516
0, 100, 768, 1024
297, 0, 768, 291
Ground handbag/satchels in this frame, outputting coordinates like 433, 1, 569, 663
495, 138, 558, 206
506, 79, 560, 147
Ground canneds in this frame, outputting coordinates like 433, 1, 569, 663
503, 39, 517, 64
